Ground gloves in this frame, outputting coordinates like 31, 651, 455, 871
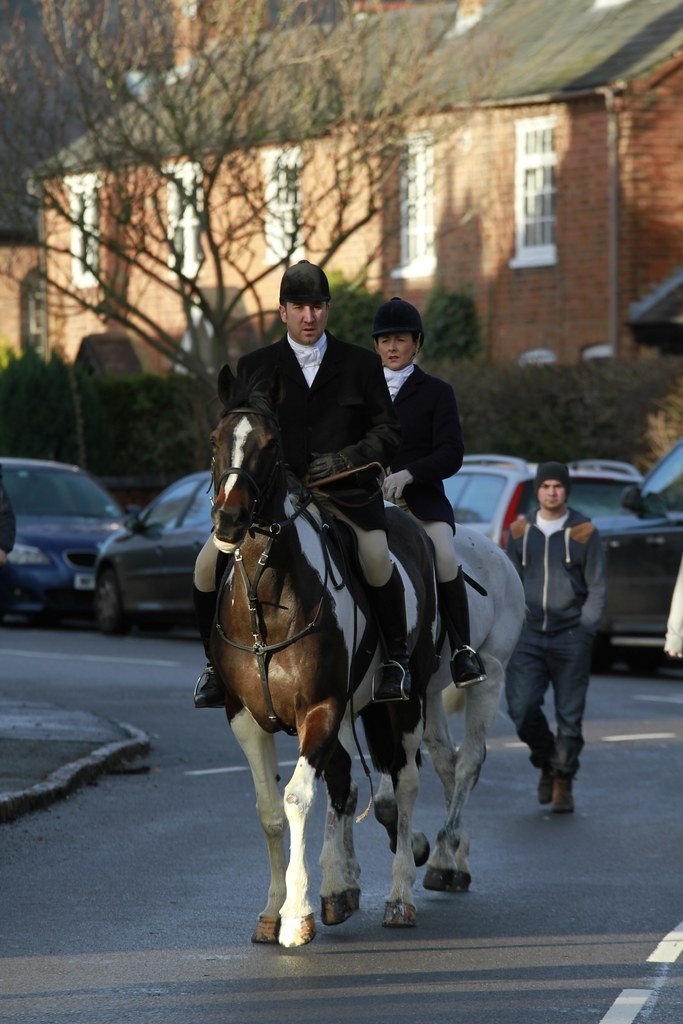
308, 452, 353, 478
382, 469, 414, 499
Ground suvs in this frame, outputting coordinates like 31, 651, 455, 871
445, 455, 646, 555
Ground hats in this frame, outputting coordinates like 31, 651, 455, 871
534, 461, 568, 499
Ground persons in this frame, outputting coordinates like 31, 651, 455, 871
194, 260, 410, 704
371, 298, 481, 680
505, 461, 606, 812
0, 464, 16, 564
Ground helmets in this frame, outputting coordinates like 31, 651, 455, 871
371, 297, 422, 338
279, 260, 330, 305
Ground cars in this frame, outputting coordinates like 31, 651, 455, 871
91, 469, 215, 638
0, 456, 154, 636
591, 434, 683, 670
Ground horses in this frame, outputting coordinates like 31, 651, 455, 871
202, 366, 530, 946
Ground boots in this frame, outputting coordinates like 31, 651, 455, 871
192, 583, 226, 708
361, 562, 412, 700
436, 564, 480, 682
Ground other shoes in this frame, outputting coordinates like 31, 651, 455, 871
539, 768, 554, 803
552, 779, 574, 813
